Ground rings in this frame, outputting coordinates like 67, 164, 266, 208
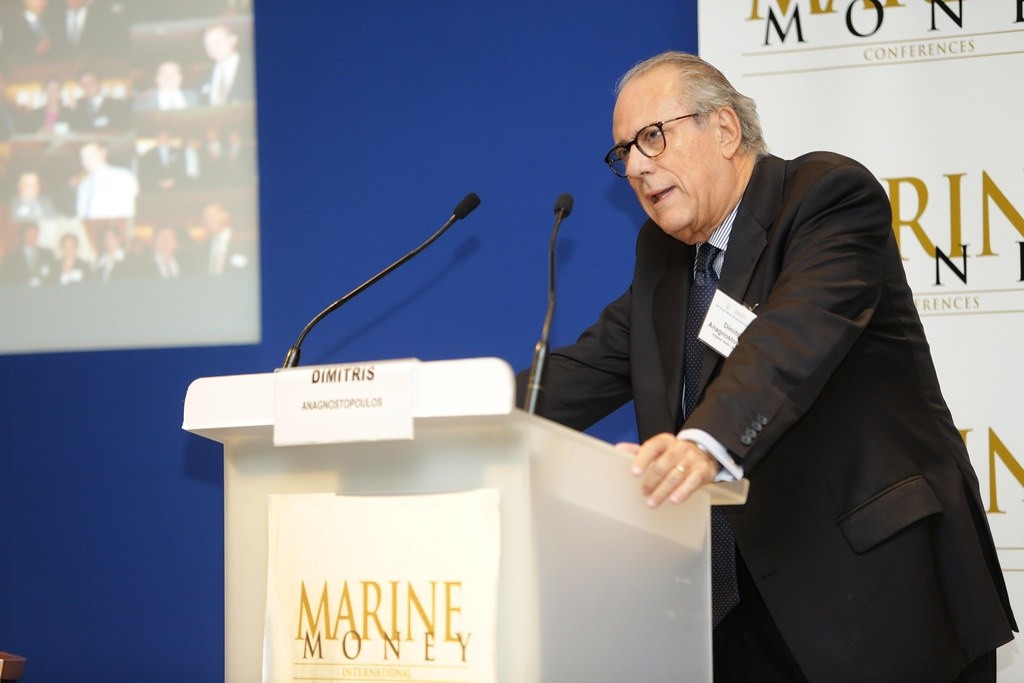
675, 464, 688, 473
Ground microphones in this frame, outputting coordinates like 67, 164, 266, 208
284, 191, 481, 367
523, 191, 574, 412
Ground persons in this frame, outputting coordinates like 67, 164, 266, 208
0, 0, 255, 300
514, 51, 1019, 683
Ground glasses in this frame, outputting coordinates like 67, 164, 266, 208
604, 112, 709, 179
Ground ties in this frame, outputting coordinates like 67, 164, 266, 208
684, 241, 739, 632
